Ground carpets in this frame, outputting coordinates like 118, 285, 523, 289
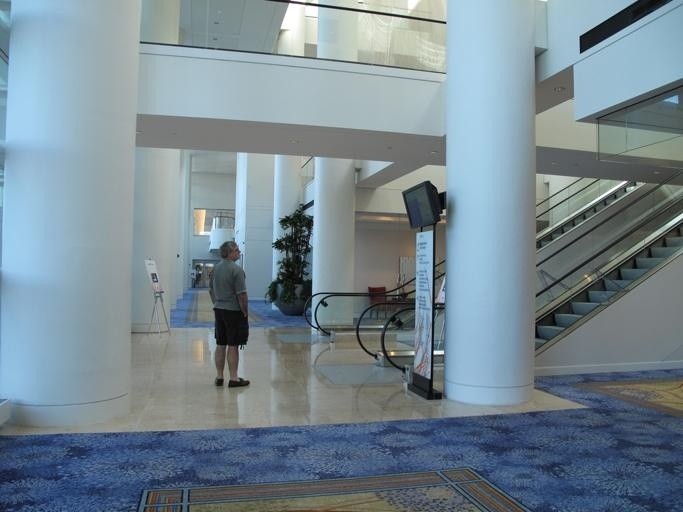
534, 368, 683, 407
0, 407, 683, 511
170, 286, 312, 329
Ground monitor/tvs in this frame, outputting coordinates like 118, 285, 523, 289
401, 180, 443, 229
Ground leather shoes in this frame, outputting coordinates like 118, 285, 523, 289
215, 377, 249, 387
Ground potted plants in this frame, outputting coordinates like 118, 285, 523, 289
263, 203, 313, 316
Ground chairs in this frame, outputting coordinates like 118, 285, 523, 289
368, 287, 387, 320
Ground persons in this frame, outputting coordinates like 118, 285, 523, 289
215, 385, 248, 429
207, 240, 249, 389
